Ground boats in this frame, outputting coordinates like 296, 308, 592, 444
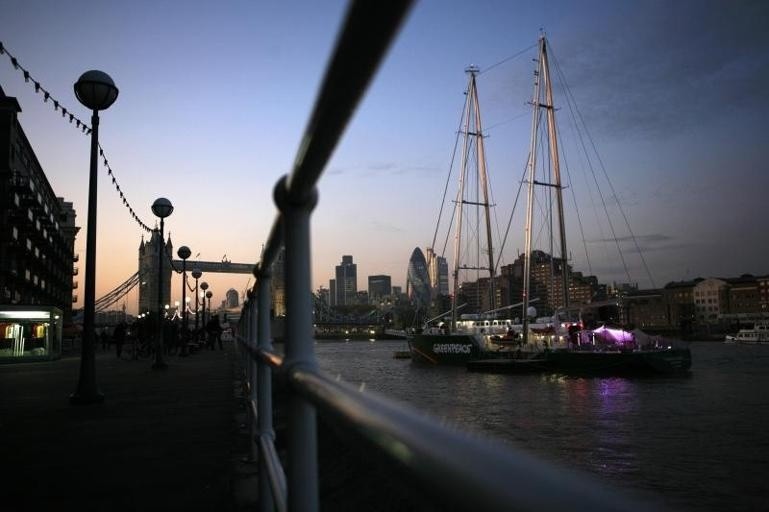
393, 349, 414, 359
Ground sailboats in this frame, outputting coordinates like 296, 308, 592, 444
407, 29, 693, 377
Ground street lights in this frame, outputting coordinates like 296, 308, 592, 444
73, 68, 119, 401
176, 243, 213, 350
148, 195, 174, 358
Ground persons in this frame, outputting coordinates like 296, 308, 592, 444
101, 326, 111, 352
205, 316, 214, 349
113, 323, 127, 359
211, 314, 223, 350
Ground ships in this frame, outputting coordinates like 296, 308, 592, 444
724, 322, 769, 346
